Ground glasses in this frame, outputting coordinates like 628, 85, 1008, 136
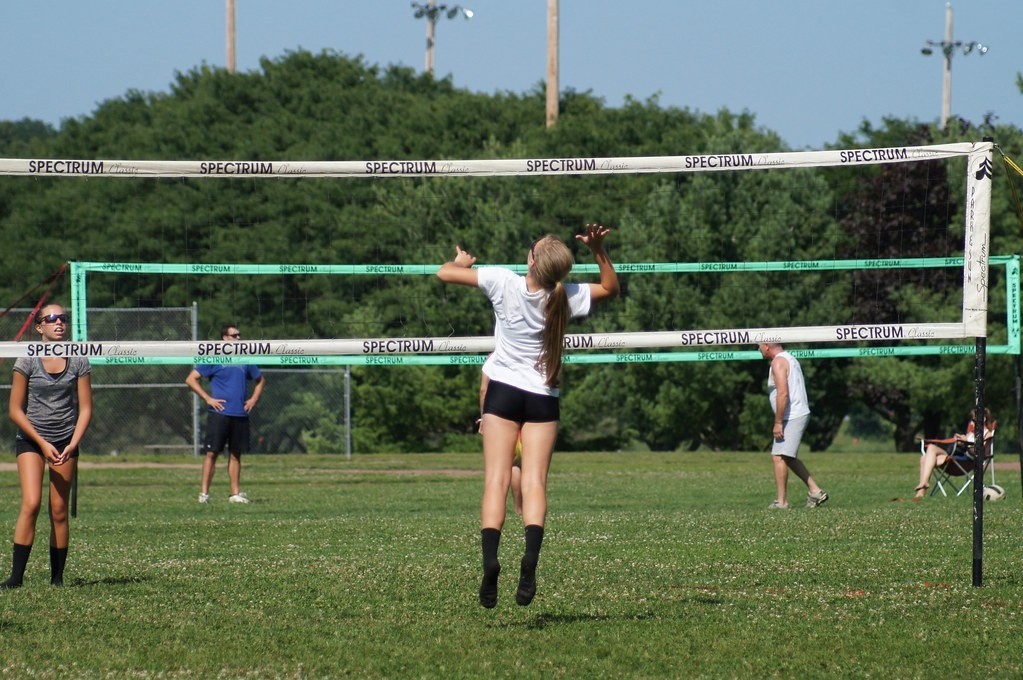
36, 314, 68, 324
227, 334, 240, 338
530, 238, 542, 260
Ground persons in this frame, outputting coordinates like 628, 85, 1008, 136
913, 407, 993, 497
437, 223, 620, 609
756, 343, 829, 509
0, 303, 93, 593
185, 325, 265, 504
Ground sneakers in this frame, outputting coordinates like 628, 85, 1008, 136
767, 500, 789, 509
805, 489, 828, 509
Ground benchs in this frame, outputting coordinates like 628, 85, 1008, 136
144, 443, 229, 459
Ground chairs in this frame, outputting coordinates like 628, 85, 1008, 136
913, 419, 998, 499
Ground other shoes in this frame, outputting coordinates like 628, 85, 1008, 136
198, 492, 207, 503
229, 495, 248, 504
